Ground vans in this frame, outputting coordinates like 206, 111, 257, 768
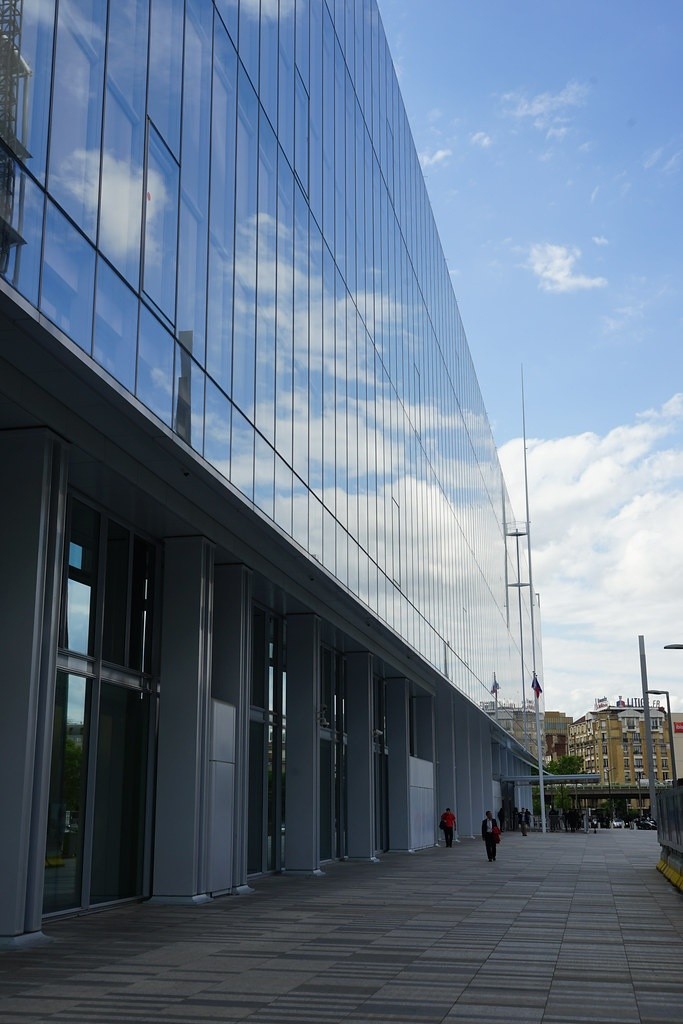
637, 778, 674, 788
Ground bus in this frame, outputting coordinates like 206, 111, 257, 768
573, 807, 612, 828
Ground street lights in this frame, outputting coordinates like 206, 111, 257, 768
645, 689, 677, 787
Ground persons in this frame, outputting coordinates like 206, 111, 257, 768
512, 808, 531, 836
561, 806, 581, 832
441, 808, 457, 848
498, 808, 505, 832
482, 811, 501, 862
549, 808, 562, 832
591, 810, 651, 827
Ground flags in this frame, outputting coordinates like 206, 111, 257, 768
532, 679, 542, 697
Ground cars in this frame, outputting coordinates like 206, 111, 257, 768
633, 816, 659, 830
609, 818, 625, 829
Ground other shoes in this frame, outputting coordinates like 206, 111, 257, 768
450, 845, 452, 848
446, 845, 449, 847
493, 857, 495, 860
522, 834, 527, 836
489, 859, 492, 862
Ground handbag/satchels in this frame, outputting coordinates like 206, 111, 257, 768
439, 821, 444, 829
496, 834, 500, 843
